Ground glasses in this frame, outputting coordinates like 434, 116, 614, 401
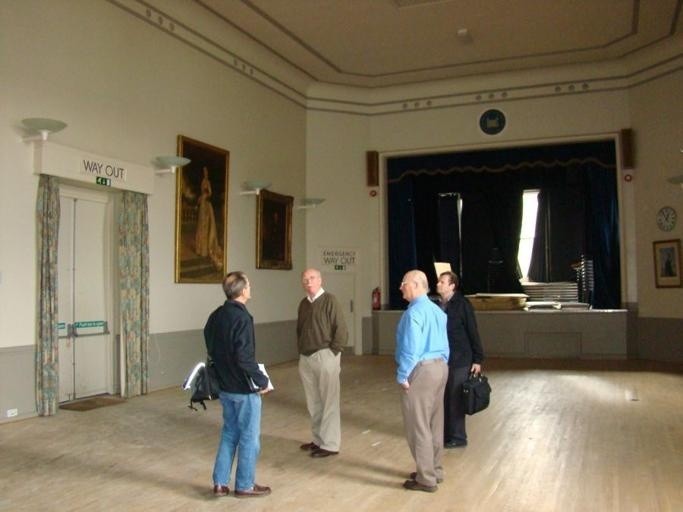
402, 280, 417, 286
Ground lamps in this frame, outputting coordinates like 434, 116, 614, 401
153, 156, 192, 174
237, 178, 273, 196
16, 117, 68, 144
293, 198, 330, 210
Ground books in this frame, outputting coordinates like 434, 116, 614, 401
245, 362, 275, 392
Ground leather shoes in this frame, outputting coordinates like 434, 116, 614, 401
300, 441, 322, 451
444, 439, 468, 448
404, 480, 438, 493
212, 485, 230, 497
410, 471, 443, 483
311, 447, 339, 458
233, 484, 272, 498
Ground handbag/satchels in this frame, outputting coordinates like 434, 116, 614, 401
460, 369, 493, 416
187, 364, 220, 413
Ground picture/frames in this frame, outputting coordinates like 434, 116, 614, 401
254, 188, 293, 269
173, 133, 230, 284
650, 238, 682, 289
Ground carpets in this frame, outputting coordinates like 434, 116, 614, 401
58, 395, 129, 412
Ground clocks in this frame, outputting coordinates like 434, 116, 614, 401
654, 205, 678, 233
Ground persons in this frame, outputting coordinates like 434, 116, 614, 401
193, 164, 219, 258
295, 267, 350, 460
660, 250, 675, 276
434, 270, 484, 449
394, 269, 451, 493
203, 269, 271, 498
269, 210, 285, 259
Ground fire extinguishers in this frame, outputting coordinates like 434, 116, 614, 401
372, 286, 381, 311
